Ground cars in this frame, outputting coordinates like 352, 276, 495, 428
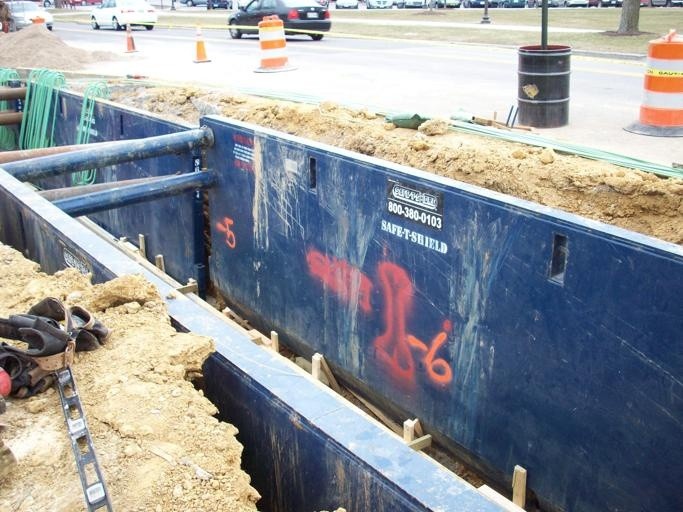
0, 1, 54, 32
88, 0, 160, 31
40, 0, 102, 9
180, 0, 246, 10
225, 0, 332, 41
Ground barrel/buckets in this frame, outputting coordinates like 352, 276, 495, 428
518, 44, 570, 128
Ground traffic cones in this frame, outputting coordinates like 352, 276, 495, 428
122, 23, 136, 54
192, 27, 210, 64
